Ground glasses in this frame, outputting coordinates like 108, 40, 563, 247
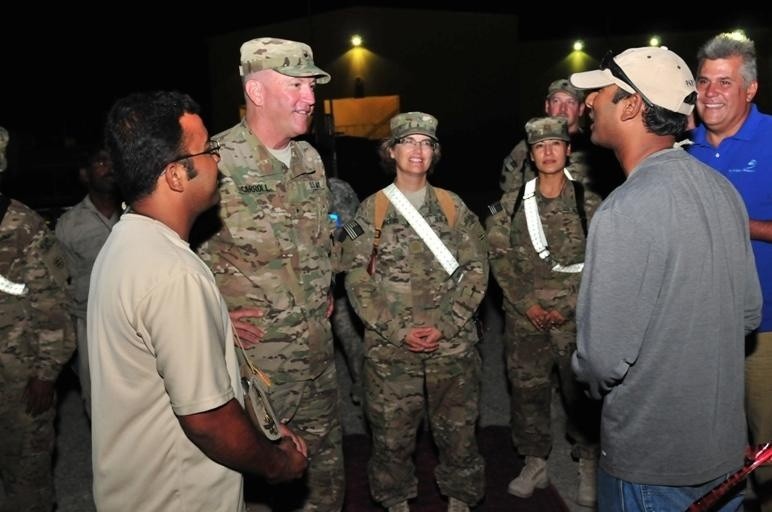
173, 140, 220, 160
599, 50, 655, 108
400, 138, 433, 146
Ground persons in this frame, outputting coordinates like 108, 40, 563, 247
1, 32, 771, 512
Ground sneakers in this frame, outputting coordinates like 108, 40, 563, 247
578, 458, 598, 506
448, 497, 470, 512
508, 458, 548, 498
389, 500, 410, 512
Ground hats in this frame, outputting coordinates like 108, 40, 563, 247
568, 46, 699, 116
547, 79, 584, 101
525, 116, 569, 144
390, 112, 439, 141
239, 37, 332, 85
